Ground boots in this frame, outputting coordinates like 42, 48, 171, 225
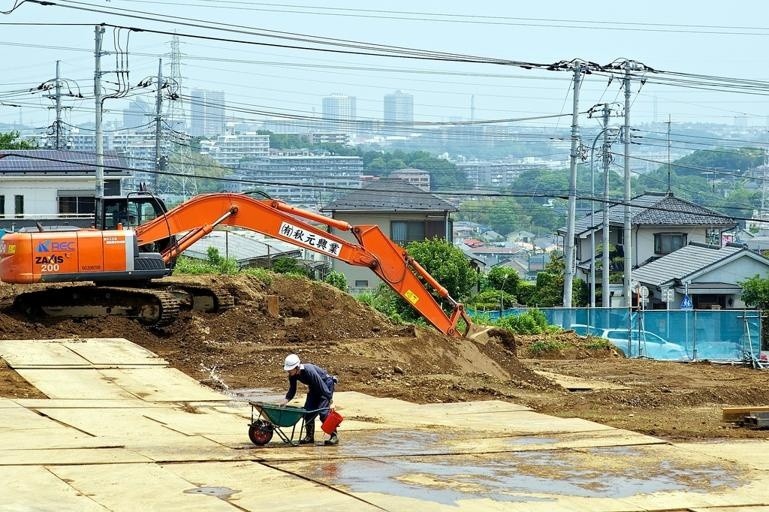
325, 428, 339, 444
300, 423, 314, 444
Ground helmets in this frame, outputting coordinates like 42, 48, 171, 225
283, 354, 301, 371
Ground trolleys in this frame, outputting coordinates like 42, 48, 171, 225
248, 401, 336, 447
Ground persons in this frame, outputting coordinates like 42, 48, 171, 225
277, 352, 339, 444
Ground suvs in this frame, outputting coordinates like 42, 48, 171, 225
569, 322, 687, 359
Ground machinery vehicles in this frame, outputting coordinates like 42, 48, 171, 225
0, 192, 517, 359
